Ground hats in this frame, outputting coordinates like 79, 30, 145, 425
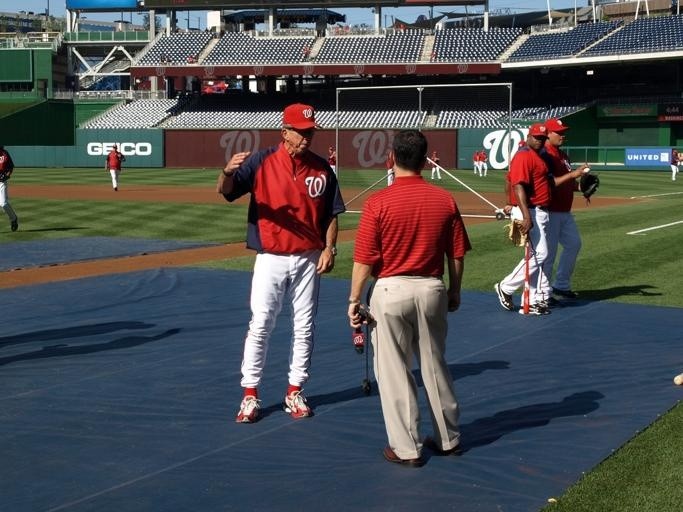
545, 118, 568, 139
283, 104, 322, 130
529, 123, 550, 140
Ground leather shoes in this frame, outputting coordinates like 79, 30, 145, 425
383, 434, 464, 468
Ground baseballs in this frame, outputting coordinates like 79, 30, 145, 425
583, 167, 590, 172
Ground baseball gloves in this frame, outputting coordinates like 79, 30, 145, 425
508, 219, 528, 246
579, 175, 599, 198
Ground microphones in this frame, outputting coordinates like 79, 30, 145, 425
353, 304, 365, 354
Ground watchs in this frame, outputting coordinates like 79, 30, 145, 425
326, 243, 338, 255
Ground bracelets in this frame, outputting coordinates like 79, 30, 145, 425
222, 169, 234, 177
349, 299, 360, 304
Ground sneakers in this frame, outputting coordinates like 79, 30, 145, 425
11, 217, 17, 232
235, 395, 263, 423
281, 389, 312, 419
494, 283, 578, 315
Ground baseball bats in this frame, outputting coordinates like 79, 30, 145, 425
523, 242, 529, 314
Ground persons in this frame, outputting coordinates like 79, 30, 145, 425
104, 143, 126, 192
670, 148, 681, 183
316, 21, 324, 37
490, 122, 556, 316
428, 150, 442, 180
348, 128, 472, 467
472, 147, 481, 175
479, 149, 489, 177
0, 139, 19, 232
328, 143, 337, 175
216, 102, 346, 425
386, 151, 395, 187
539, 115, 591, 302
